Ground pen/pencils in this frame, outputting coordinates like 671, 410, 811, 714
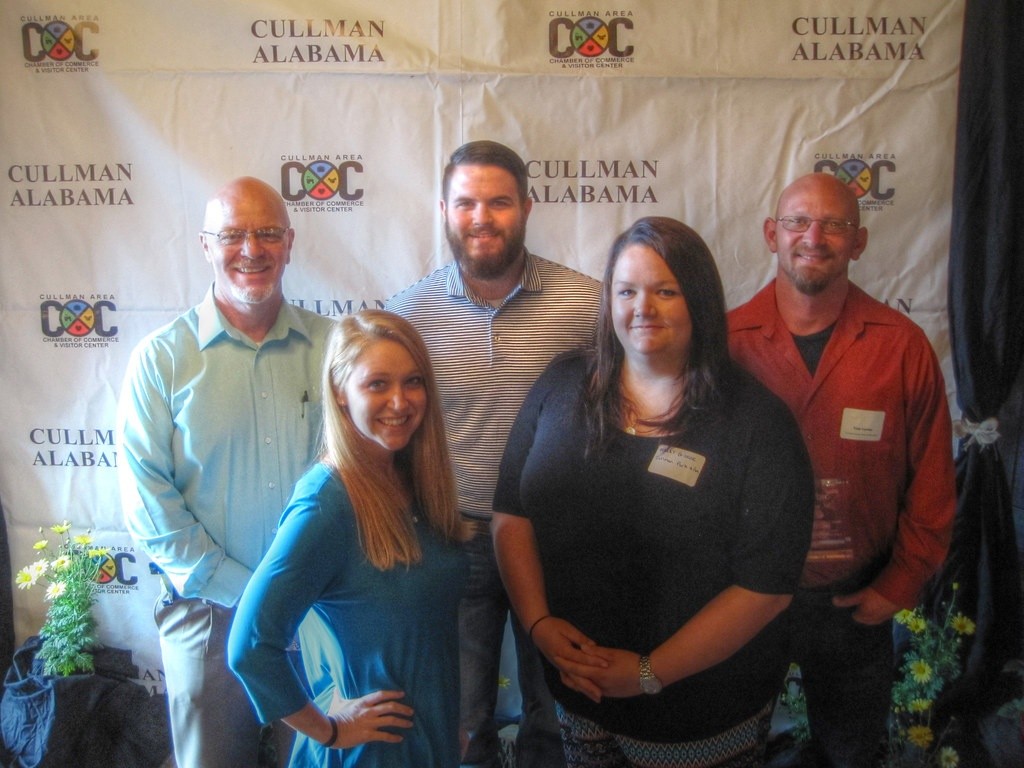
300, 390, 308, 418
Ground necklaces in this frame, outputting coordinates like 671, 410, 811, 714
624, 425, 636, 435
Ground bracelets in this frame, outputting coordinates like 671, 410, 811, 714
529, 615, 550, 640
323, 713, 338, 747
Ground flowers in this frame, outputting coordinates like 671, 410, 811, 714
784, 579, 976, 768
16, 520, 112, 676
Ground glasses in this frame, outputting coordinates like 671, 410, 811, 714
776, 214, 858, 235
204, 226, 288, 247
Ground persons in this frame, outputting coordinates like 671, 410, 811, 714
127, 178, 341, 767
490, 217, 814, 768
229, 309, 482, 767
386, 140, 607, 768
729, 176, 955, 768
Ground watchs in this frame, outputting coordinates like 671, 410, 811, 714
639, 651, 663, 694
